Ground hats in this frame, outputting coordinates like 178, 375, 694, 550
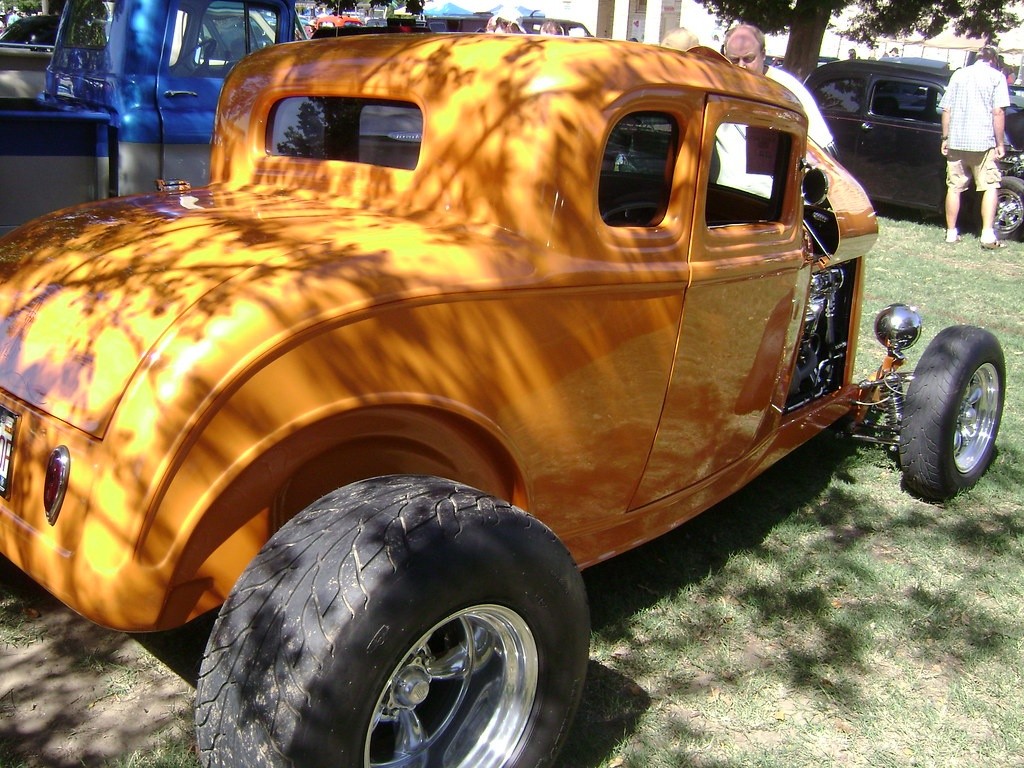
889, 48, 898, 53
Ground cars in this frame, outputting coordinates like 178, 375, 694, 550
800, 58, 1024, 244
316, 16, 365, 34
0, 18, 1009, 768
1, 13, 60, 46
764, 54, 786, 69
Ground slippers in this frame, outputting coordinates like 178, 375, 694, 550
946, 235, 963, 245
981, 239, 1007, 250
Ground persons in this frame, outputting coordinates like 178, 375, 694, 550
715, 25, 831, 204
541, 21, 565, 35
883, 47, 899, 57
939, 45, 1016, 249
848, 49, 856, 59
487, 17, 521, 34
658, 27, 700, 52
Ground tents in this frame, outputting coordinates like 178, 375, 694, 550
832, 0, 1024, 80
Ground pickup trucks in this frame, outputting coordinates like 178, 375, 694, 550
0, 1, 315, 245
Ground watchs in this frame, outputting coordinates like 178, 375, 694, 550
941, 135, 948, 140
